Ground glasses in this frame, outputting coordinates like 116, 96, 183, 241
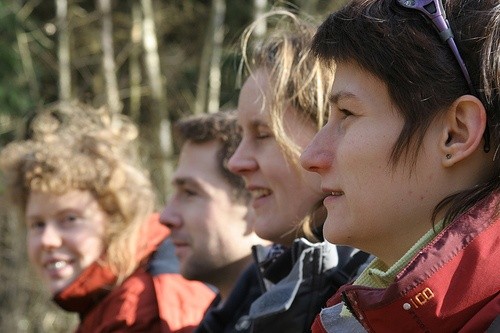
401, 0, 491, 153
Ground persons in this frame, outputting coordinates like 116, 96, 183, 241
158, 0, 500, 333
0, 101, 220, 333
